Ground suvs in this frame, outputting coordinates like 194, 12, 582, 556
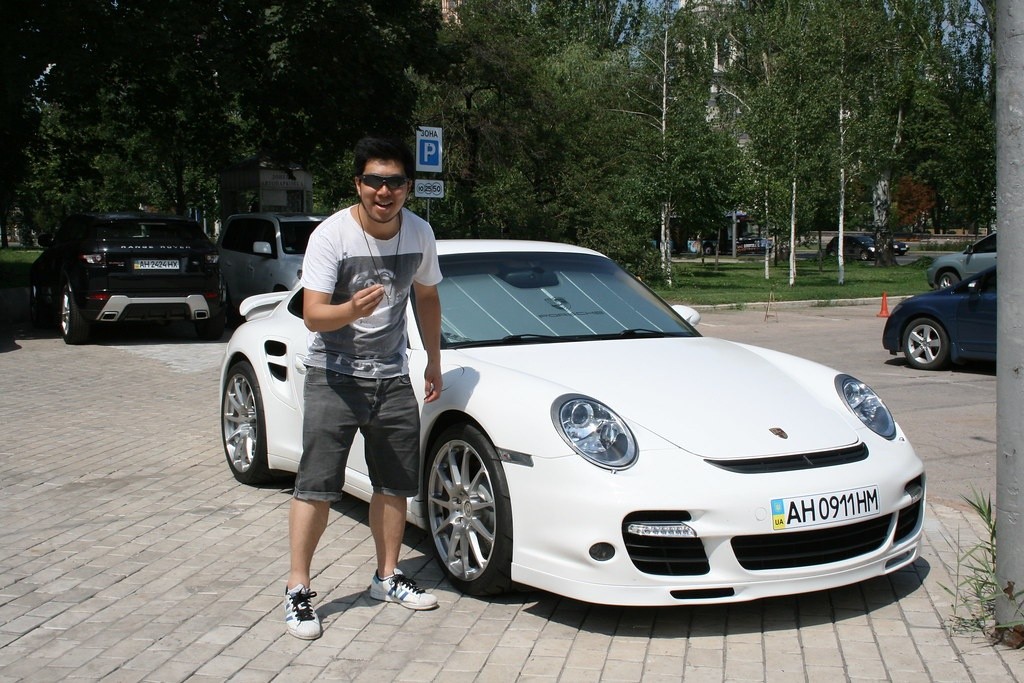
217, 213, 329, 322
28, 213, 229, 344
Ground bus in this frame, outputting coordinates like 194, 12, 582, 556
653, 211, 749, 256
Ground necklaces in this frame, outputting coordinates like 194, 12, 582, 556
357, 201, 402, 308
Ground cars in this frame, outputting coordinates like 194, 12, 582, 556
826, 236, 876, 261
721, 238, 758, 256
927, 231, 998, 291
865, 234, 910, 256
751, 238, 773, 254
882, 264, 997, 370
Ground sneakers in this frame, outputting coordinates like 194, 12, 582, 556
283, 585, 321, 640
369, 569, 438, 610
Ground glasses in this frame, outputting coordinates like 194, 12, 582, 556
359, 174, 409, 189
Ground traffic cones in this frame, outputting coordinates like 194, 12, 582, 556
877, 291, 889, 317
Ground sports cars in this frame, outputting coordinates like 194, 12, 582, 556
218, 237, 928, 606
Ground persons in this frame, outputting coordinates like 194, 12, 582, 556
282, 135, 445, 640
